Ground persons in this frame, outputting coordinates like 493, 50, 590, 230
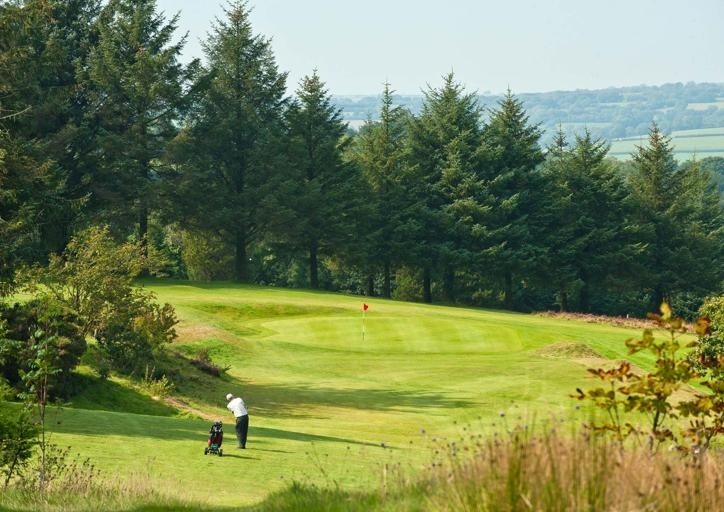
226, 393, 249, 449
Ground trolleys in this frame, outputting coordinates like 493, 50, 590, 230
204, 422, 224, 457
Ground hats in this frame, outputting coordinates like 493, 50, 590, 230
226, 393, 233, 400
